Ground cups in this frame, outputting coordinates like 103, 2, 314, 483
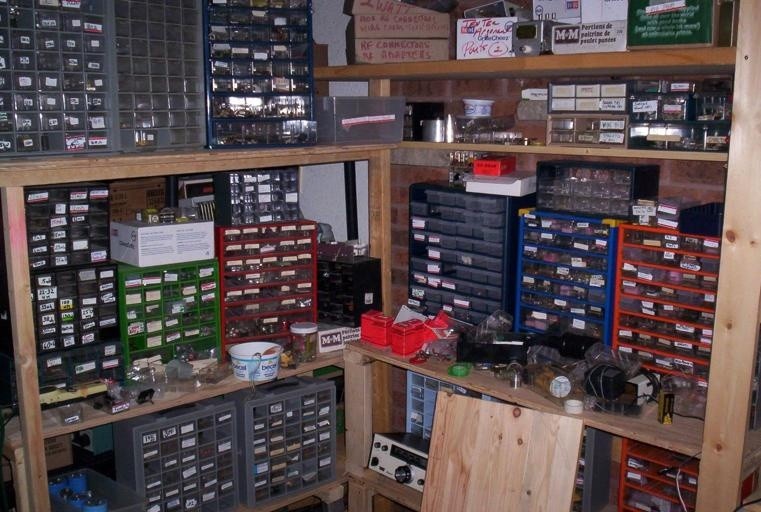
460, 97, 494, 118
422, 119, 447, 143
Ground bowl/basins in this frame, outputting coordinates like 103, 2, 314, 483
227, 341, 285, 382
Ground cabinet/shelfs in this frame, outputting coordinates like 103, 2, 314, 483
2, 2, 760, 512
